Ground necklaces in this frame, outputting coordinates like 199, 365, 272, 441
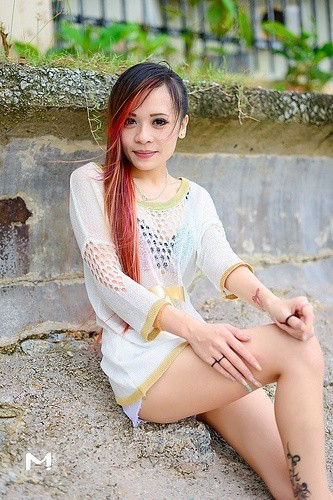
132, 168, 169, 200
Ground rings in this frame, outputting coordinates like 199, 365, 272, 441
285, 314, 296, 323
218, 356, 224, 363
301, 303, 312, 311
211, 360, 217, 367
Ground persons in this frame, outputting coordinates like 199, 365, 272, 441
67, 61, 333, 500
